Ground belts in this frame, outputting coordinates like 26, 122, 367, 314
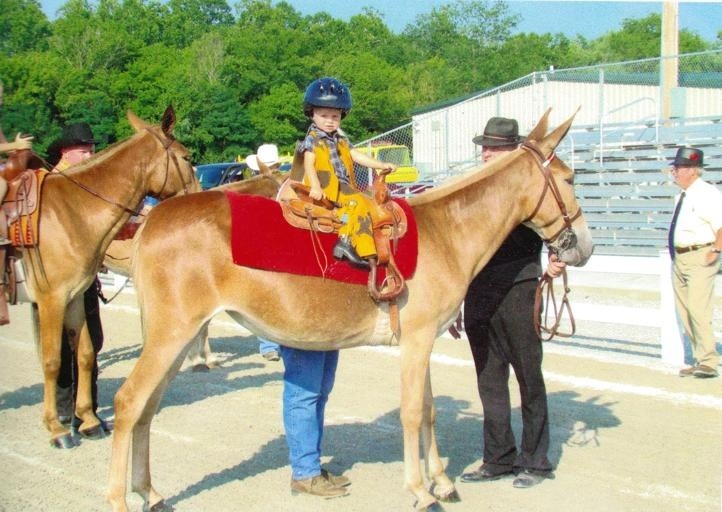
676, 243, 712, 253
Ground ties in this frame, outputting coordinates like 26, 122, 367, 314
669, 193, 685, 260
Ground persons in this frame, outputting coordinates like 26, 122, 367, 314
287, 78, 396, 268
274, 346, 352, 500
447, 118, 566, 489
668, 147, 722, 377
246, 144, 282, 362
0, 129, 34, 155
33, 122, 113, 431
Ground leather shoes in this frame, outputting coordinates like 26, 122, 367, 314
290, 468, 352, 498
460, 463, 509, 481
333, 241, 368, 265
680, 366, 716, 376
512, 470, 545, 487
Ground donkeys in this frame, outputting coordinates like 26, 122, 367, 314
99, 104, 596, 512
101, 155, 293, 373
0, 103, 205, 450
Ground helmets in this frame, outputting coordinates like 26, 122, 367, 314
304, 77, 352, 119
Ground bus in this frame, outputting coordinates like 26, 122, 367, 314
236, 139, 420, 186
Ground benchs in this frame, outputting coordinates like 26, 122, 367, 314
428, 118, 719, 255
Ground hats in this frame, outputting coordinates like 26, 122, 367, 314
473, 118, 527, 146
245, 144, 291, 171
668, 148, 708, 166
48, 122, 100, 154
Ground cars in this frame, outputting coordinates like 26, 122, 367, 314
194, 159, 245, 194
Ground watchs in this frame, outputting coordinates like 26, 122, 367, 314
710, 247, 720, 253
